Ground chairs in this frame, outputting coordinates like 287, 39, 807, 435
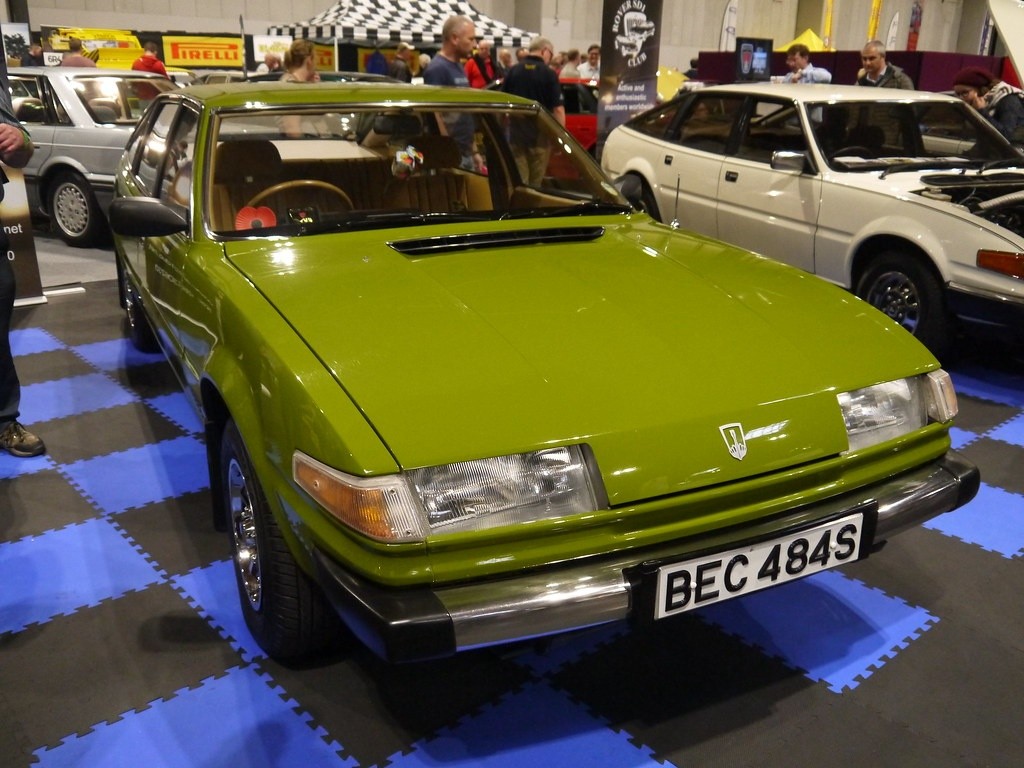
204, 135, 290, 231
373, 135, 493, 215
10, 97, 42, 122
88, 97, 121, 122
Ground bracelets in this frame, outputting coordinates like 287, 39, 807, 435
797, 68, 803, 76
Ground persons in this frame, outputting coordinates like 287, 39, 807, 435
20, 45, 45, 95
464, 40, 505, 132
498, 48, 511, 74
276, 39, 339, 182
414, 53, 431, 78
770, 44, 831, 126
952, 66, 1024, 231
846, 40, 918, 148
130, 41, 171, 114
502, 33, 564, 189
549, 51, 568, 75
0, 76, 49, 458
575, 43, 600, 99
421, 15, 478, 214
386, 42, 412, 84
257, 52, 284, 74
355, 111, 410, 147
60, 37, 97, 68
516, 47, 530, 63
559, 48, 581, 114
682, 58, 698, 80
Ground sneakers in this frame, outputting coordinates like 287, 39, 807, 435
0, 422, 46, 457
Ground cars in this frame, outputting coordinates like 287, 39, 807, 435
6, 66, 182, 247
165, 70, 405, 89
601, 83, 1024, 358
108, 81, 981, 668
483, 77, 662, 165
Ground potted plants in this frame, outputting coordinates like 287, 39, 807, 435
4, 33, 25, 66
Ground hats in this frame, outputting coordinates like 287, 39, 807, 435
397, 42, 415, 51
953, 68, 993, 87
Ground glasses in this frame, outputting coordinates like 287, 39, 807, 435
955, 89, 974, 98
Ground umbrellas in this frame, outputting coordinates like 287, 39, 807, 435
267, 0, 540, 53
773, 28, 837, 51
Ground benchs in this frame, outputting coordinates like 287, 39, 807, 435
170, 157, 435, 210
